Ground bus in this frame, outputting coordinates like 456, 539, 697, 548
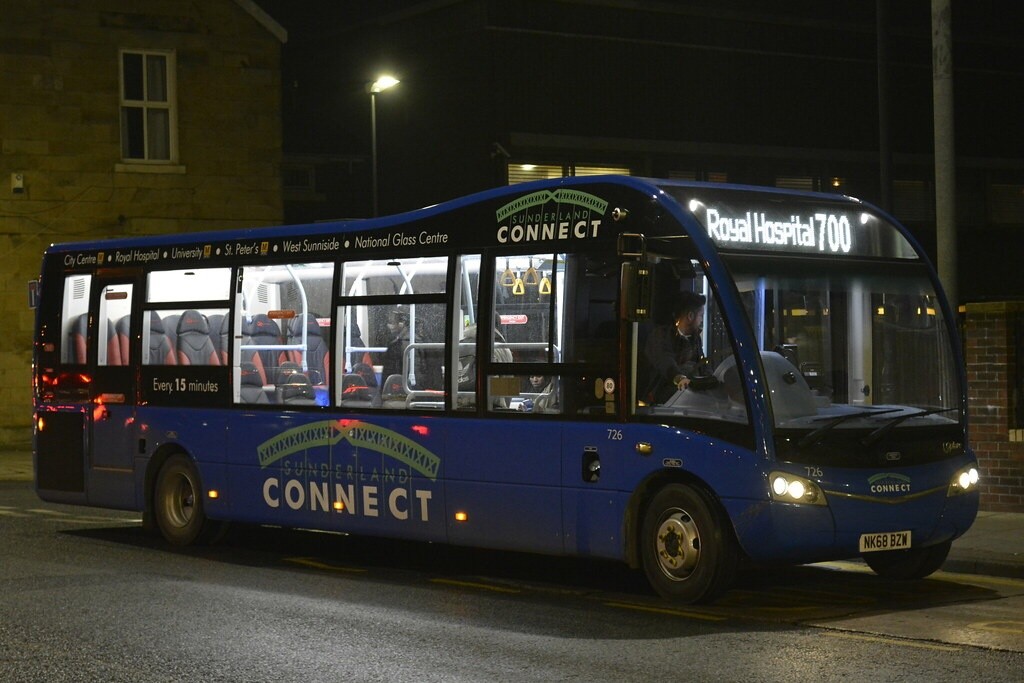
30, 174, 984, 609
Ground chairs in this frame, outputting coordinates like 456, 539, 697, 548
72, 310, 411, 410
649, 299, 674, 326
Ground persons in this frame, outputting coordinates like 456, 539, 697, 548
458, 311, 551, 407
377, 310, 427, 393
637, 291, 706, 407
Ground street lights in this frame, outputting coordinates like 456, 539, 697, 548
368, 74, 402, 217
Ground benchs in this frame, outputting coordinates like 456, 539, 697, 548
403, 343, 559, 414
